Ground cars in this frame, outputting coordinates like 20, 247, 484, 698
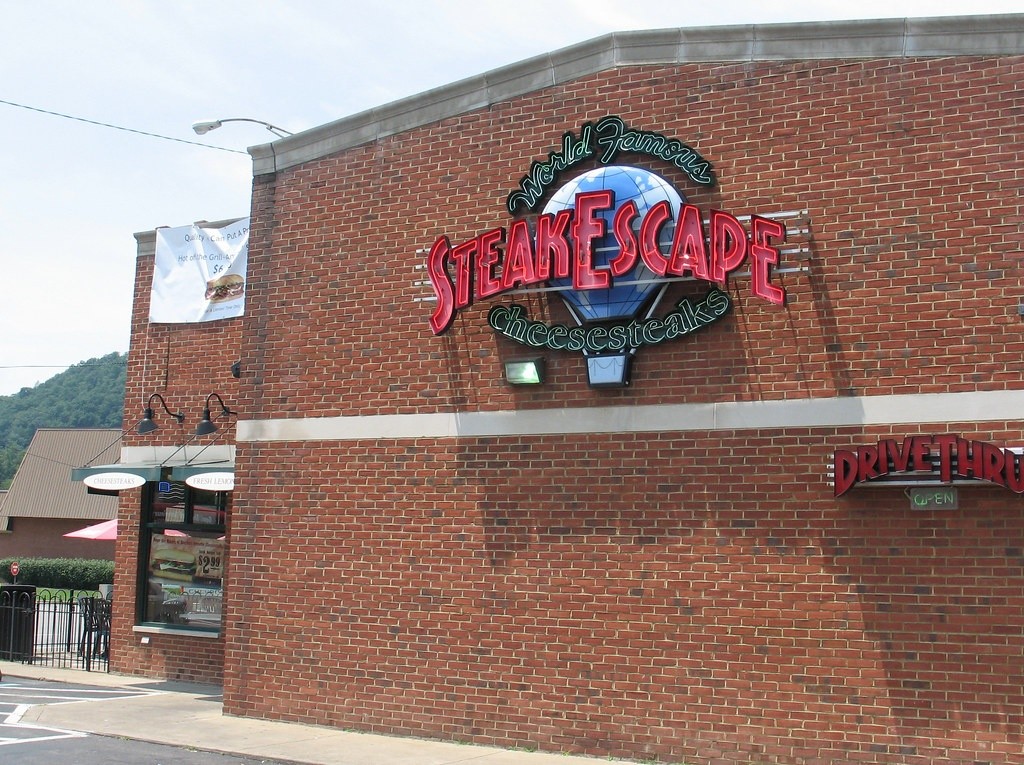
144, 578, 189, 623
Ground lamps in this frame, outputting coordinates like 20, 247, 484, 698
505, 357, 546, 387
192, 119, 293, 139
583, 352, 634, 388
138, 392, 185, 434
196, 393, 238, 436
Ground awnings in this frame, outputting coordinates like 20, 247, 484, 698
67, 452, 236, 489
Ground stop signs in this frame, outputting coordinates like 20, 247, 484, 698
11, 562, 19, 575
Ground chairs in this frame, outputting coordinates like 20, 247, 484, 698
81, 596, 112, 659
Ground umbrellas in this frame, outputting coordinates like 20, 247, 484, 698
62, 516, 192, 542
